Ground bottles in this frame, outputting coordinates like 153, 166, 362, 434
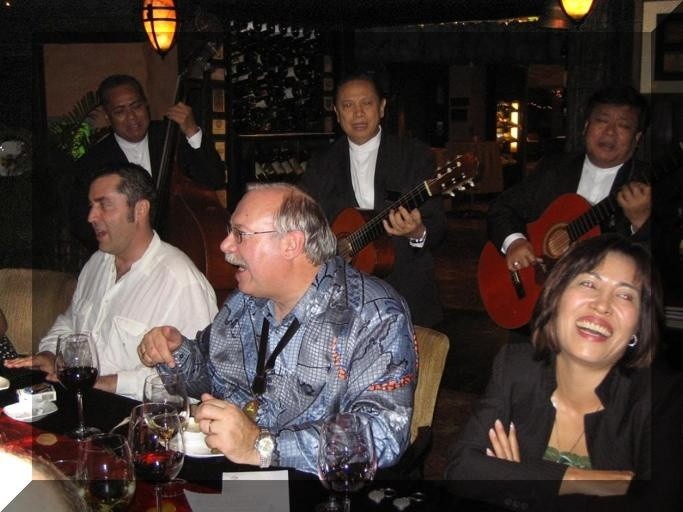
251, 145, 308, 178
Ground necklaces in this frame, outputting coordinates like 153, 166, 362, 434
545, 390, 604, 466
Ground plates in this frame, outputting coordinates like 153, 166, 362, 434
5, 399, 61, 425
158, 416, 224, 458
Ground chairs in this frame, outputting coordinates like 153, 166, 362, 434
409, 324, 450, 446
1, 268, 63, 357
55, 277, 78, 318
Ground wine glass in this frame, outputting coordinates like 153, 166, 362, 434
141, 371, 192, 434
53, 332, 107, 444
315, 412, 376, 511
128, 402, 187, 504
76, 430, 139, 508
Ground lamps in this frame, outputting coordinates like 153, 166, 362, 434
142, 0, 181, 62
558, 0, 597, 31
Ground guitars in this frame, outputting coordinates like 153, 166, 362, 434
478, 141, 682, 329
330, 152, 481, 282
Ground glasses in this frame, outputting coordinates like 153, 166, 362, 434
224, 224, 284, 244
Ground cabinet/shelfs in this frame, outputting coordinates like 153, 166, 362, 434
445, 83, 574, 181
202, 29, 337, 209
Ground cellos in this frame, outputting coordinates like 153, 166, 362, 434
153, 34, 239, 311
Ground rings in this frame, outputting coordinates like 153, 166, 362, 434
513, 261, 520, 266
140, 350, 148, 359
502, 457, 507, 460
205, 418, 217, 435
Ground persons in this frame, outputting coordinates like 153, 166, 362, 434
135, 178, 421, 479
3, 160, 223, 409
72, 71, 232, 256
481, 86, 683, 348
286, 72, 450, 334
444, 232, 681, 511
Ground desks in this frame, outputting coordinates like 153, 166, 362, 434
1, 364, 520, 512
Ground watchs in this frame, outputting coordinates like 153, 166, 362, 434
406, 225, 428, 245
253, 427, 278, 470
